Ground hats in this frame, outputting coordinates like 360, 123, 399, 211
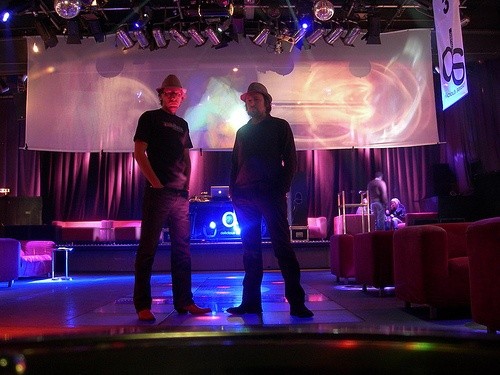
156, 74, 191, 93
240, 82, 273, 102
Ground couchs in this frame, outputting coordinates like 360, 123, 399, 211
0, 237, 55, 286
307, 215, 328, 240
327, 212, 500, 335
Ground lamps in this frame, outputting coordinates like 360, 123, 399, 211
22, 0, 386, 52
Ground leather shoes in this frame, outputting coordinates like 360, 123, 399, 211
177, 303, 211, 314
138, 310, 155, 321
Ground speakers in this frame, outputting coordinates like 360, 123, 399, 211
435, 171, 461, 209
0, 195, 43, 225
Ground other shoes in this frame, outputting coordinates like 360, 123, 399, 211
227, 303, 264, 314
290, 307, 314, 318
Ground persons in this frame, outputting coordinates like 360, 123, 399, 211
226, 82, 315, 318
356, 171, 405, 230
133, 74, 213, 323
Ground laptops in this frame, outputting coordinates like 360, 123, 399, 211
210, 186, 230, 199
